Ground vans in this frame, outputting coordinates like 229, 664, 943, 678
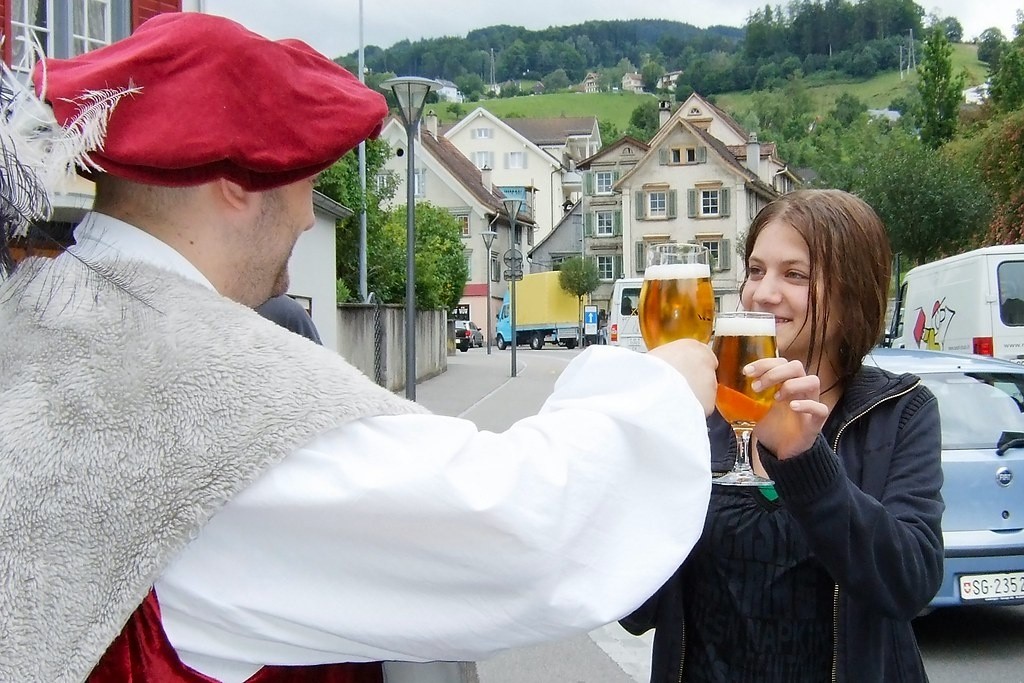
880, 244, 1024, 409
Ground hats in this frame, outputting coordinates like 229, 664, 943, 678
30, 10, 388, 190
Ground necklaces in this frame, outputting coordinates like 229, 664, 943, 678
819, 381, 840, 396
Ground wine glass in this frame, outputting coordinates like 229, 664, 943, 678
707, 312, 779, 486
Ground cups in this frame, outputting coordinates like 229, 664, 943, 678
640, 244, 713, 349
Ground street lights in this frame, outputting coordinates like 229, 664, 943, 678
499, 198, 527, 378
478, 230, 498, 354
377, 75, 444, 403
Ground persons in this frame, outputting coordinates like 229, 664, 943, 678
618, 189, 951, 682
2, 9, 720, 683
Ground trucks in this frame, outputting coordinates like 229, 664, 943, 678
596, 273, 649, 354
494, 269, 590, 351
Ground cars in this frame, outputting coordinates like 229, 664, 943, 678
858, 343, 1024, 619
463, 320, 484, 348
454, 320, 470, 352
